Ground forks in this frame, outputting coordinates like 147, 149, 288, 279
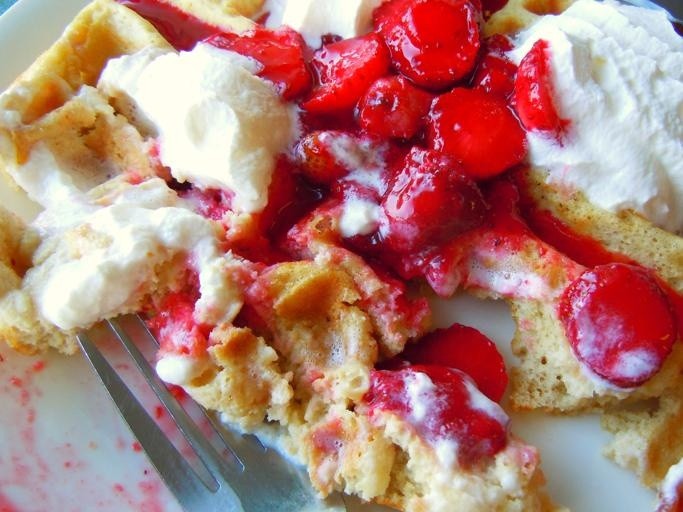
72, 308, 352, 512
